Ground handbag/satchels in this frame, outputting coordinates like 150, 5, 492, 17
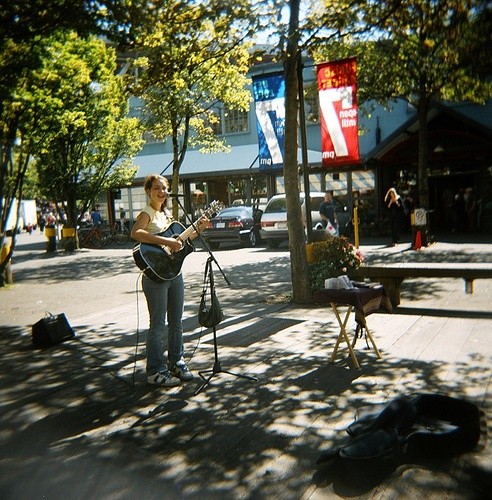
198, 260, 224, 328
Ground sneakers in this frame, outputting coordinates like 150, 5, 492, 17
147, 369, 180, 386
170, 361, 193, 380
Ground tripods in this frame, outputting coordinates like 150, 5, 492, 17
173, 197, 257, 395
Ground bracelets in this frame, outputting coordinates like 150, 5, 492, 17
199, 227, 204, 231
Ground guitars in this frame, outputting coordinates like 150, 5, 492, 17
133, 200, 224, 284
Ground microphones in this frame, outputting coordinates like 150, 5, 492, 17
165, 192, 186, 197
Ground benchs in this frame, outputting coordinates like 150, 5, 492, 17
352, 261, 492, 310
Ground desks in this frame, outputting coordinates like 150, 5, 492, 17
320, 282, 384, 372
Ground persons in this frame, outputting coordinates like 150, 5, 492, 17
38, 206, 125, 233
130, 174, 210, 386
320, 187, 481, 250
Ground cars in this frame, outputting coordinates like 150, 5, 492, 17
201, 206, 268, 250
259, 191, 339, 249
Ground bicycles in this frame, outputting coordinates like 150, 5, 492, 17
82, 219, 131, 247
